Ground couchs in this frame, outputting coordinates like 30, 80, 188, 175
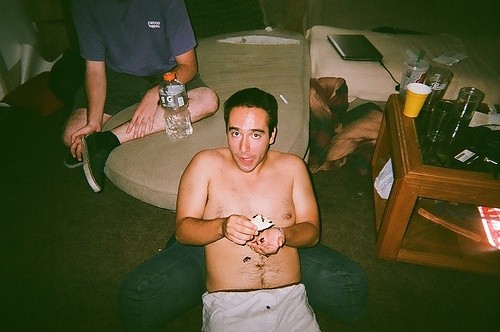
105, 0, 500, 211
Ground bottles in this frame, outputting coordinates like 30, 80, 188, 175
159, 72, 193, 139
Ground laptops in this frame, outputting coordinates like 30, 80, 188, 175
327, 35, 383, 61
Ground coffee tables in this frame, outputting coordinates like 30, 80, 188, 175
371, 94, 500, 275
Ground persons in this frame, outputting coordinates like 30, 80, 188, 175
61, 0, 218, 192
173, 86, 323, 332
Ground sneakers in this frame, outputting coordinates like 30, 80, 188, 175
82, 131, 109, 192
63, 151, 83, 168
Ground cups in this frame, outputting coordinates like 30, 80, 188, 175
456, 87, 483, 121
400, 59, 429, 98
427, 101, 458, 143
403, 83, 431, 118
425, 66, 454, 104
